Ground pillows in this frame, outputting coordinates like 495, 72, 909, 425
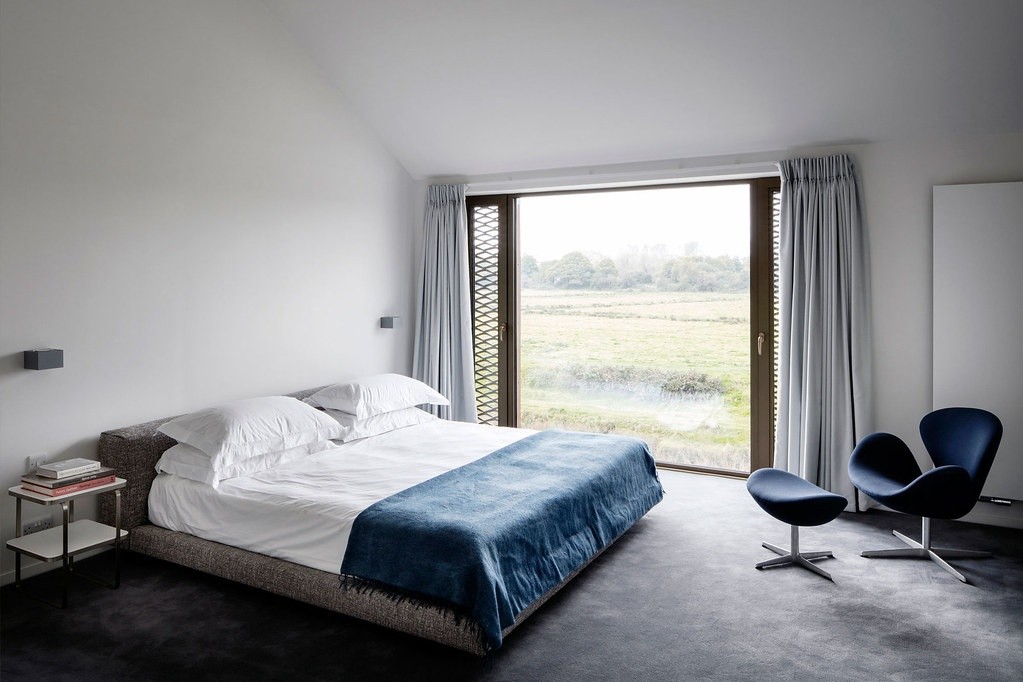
323, 407, 440, 443
154, 395, 351, 471
302, 374, 449, 421
156, 439, 339, 490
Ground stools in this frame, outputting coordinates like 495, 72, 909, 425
747, 467, 848, 580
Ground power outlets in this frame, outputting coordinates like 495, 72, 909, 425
27, 453, 47, 472
20, 515, 53, 538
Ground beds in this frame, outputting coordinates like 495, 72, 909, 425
98, 384, 666, 655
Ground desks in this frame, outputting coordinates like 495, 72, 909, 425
5, 477, 127, 610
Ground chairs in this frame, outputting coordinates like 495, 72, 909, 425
848, 407, 1003, 583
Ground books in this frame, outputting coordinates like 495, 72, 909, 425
20, 458, 116, 497
